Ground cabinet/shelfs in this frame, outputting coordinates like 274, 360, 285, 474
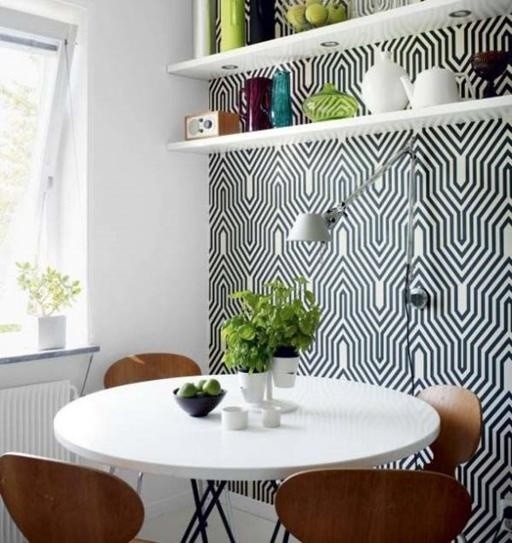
164, 0, 511, 156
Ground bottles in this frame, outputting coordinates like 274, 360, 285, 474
260, 71, 295, 128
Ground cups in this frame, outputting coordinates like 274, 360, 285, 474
221, 405, 249, 431
237, 75, 273, 131
260, 404, 283, 428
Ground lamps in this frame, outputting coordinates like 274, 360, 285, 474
283, 142, 428, 314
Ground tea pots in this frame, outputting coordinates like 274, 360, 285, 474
399, 64, 469, 110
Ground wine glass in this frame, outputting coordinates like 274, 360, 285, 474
469, 50, 509, 98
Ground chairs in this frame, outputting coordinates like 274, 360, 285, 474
416, 385, 482, 477
0, 451, 145, 543
104, 353, 202, 389
275, 468, 471, 543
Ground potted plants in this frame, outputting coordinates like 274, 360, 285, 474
218, 273, 325, 406
18, 260, 82, 351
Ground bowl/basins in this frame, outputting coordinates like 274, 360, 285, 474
171, 387, 227, 418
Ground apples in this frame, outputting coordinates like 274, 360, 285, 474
177, 379, 221, 397
288, 0, 345, 32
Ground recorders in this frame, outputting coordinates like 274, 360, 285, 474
184, 110, 240, 140
